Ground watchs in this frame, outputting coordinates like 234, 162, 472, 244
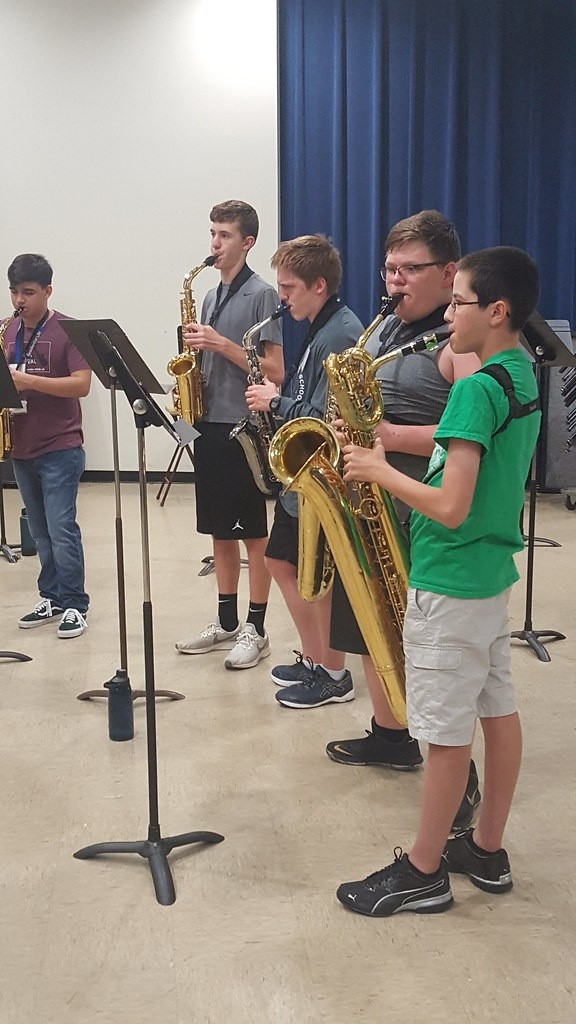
270, 396, 282, 413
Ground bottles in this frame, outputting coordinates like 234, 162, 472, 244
20, 508, 37, 556
105, 668, 134, 742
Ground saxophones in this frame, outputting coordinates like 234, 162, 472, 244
166, 256, 219, 426
281, 330, 455, 729
228, 305, 292, 496
0, 306, 26, 463
268, 292, 407, 603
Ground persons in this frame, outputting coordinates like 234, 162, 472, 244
0, 255, 93, 637
246, 236, 367, 708
336, 248, 542, 918
176, 199, 282, 669
325, 210, 481, 833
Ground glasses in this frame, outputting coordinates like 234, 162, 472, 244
379, 261, 447, 282
449, 297, 511, 318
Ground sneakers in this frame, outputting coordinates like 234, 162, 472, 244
271, 650, 316, 687
336, 847, 454, 917
275, 665, 356, 709
326, 732, 423, 771
440, 827, 514, 894
175, 615, 243, 654
451, 758, 481, 832
57, 608, 88, 637
224, 622, 271, 669
17, 598, 65, 629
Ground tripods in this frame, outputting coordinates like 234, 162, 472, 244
0, 318, 249, 905
509, 308, 576, 663
